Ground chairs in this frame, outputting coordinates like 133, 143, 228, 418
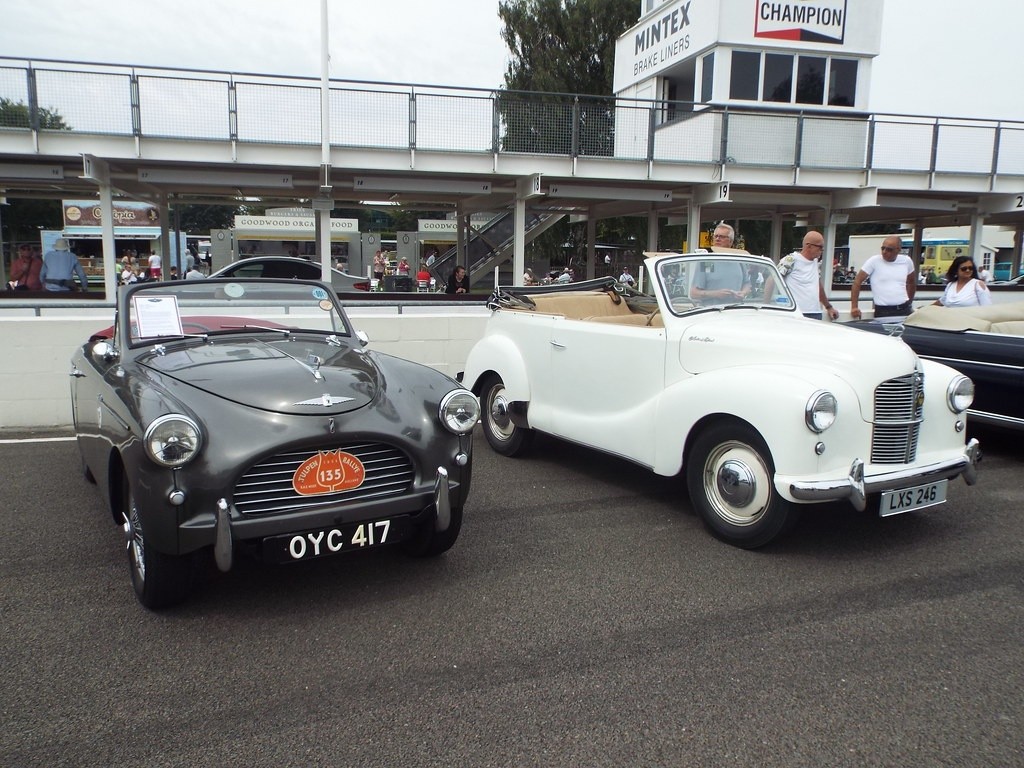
589, 315, 665, 327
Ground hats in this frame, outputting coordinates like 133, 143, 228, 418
623, 267, 628, 270
563, 267, 570, 272
402, 257, 407, 261
52, 239, 71, 251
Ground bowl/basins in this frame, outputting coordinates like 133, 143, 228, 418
90, 256, 94, 258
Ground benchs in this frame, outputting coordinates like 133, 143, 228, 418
123, 317, 287, 342
986, 320, 1024, 334
521, 293, 634, 319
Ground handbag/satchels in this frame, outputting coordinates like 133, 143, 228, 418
623, 275, 634, 287
14, 257, 34, 291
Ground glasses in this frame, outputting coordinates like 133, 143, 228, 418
806, 242, 824, 249
713, 234, 728, 239
959, 266, 973, 272
881, 246, 895, 252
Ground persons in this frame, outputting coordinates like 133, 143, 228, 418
918, 256, 992, 308
690, 223, 751, 305
763, 230, 840, 321
524, 268, 534, 285
415, 264, 431, 292
336, 263, 343, 271
425, 250, 438, 266
445, 266, 469, 294
605, 252, 611, 276
374, 250, 385, 291
149, 250, 162, 282
10, 244, 43, 293
119, 248, 144, 284
398, 257, 410, 275
544, 268, 575, 284
851, 236, 917, 321
619, 267, 636, 286
171, 248, 205, 281
39, 238, 88, 292
979, 266, 994, 283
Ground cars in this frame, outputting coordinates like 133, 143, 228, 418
462, 252, 984, 551
207, 255, 371, 291
70, 275, 482, 609
994, 262, 1024, 281
836, 304, 1024, 451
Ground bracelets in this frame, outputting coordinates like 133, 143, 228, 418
826, 304, 832, 310
